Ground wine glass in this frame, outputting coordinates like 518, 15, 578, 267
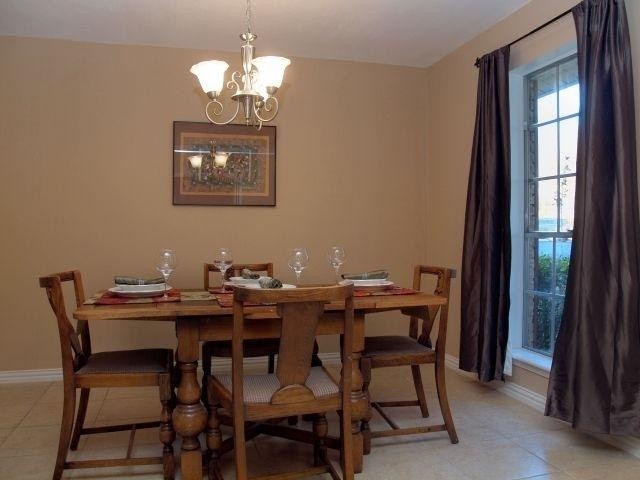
214, 248, 234, 294
327, 247, 345, 287
154, 248, 179, 303
288, 247, 309, 289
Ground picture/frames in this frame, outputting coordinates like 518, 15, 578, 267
173, 120, 277, 206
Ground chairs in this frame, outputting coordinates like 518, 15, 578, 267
41, 269, 175, 480
201, 265, 275, 407
341, 266, 457, 455
207, 281, 354, 480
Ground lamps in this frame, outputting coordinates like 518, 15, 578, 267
189, 0, 291, 131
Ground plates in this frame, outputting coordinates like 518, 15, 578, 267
339, 278, 394, 289
224, 276, 296, 290
109, 283, 172, 297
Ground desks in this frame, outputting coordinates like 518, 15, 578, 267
72, 282, 447, 480
